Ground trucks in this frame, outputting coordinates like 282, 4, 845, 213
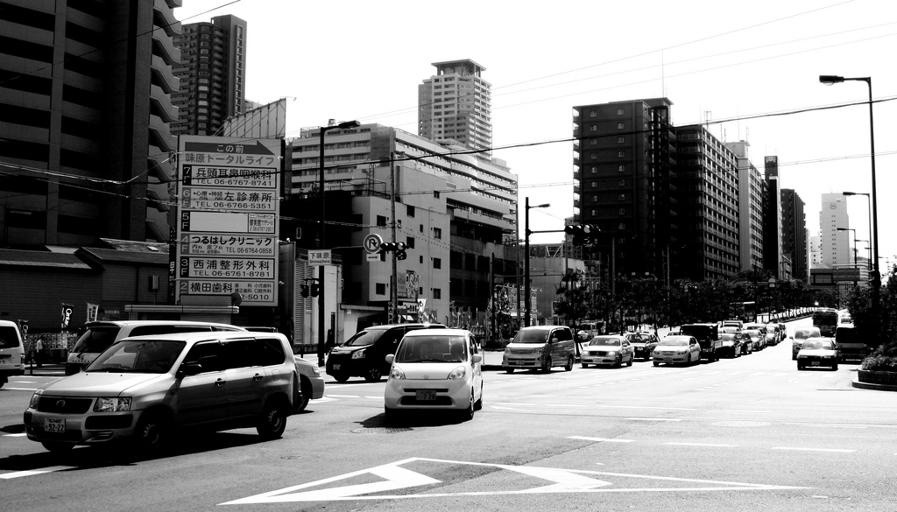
833, 324, 869, 363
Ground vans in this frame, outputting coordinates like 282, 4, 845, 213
327, 318, 449, 384
501, 324, 578, 372
0, 320, 27, 388
66, 319, 324, 411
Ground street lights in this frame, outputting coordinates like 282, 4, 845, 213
835, 226, 873, 287
818, 70, 883, 358
719, 249, 731, 319
316, 117, 361, 365
753, 256, 768, 321
842, 189, 873, 279
524, 196, 550, 329
781, 259, 787, 280
561, 273, 581, 356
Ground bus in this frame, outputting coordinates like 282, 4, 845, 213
581, 320, 609, 336
813, 307, 838, 337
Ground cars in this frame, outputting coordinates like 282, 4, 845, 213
581, 334, 635, 367
796, 338, 839, 371
384, 327, 486, 419
576, 329, 594, 341
665, 331, 683, 338
840, 312, 852, 324
681, 318, 786, 361
653, 334, 702, 367
623, 332, 660, 361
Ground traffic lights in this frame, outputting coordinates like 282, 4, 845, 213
300, 283, 309, 297
312, 284, 319, 297
380, 241, 404, 251
564, 224, 592, 235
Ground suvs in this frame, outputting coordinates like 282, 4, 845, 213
21, 331, 298, 459
789, 327, 825, 360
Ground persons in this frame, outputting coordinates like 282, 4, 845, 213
35, 338, 45, 368
